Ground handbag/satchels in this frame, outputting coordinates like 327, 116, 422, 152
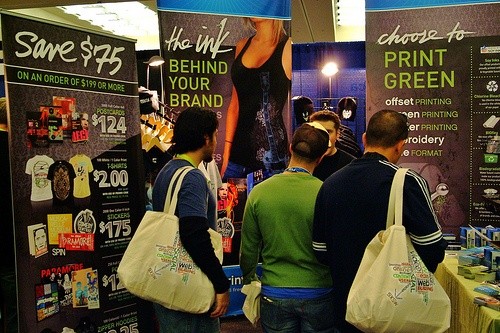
344, 166, 452, 333
117, 165, 223, 315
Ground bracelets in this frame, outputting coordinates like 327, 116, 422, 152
225, 140, 233, 143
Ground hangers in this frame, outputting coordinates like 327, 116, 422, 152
144, 102, 174, 141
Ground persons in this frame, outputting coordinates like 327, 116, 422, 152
219, 17, 292, 182
218, 183, 228, 200
310, 110, 359, 183
62, 97, 74, 116
0, 96, 19, 333
312, 109, 449, 333
48, 269, 99, 307
81, 112, 88, 129
239, 122, 340, 333
292, 95, 314, 122
151, 107, 230, 333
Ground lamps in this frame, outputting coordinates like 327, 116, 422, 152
146, 56, 165, 89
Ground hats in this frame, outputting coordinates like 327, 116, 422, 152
291, 122, 332, 160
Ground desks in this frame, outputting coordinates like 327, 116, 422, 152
436, 249, 500, 333
